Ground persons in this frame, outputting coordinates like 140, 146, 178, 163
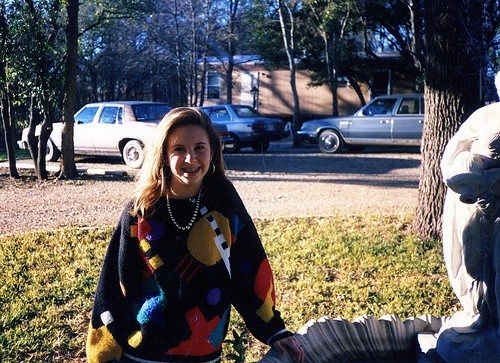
84, 106, 306, 363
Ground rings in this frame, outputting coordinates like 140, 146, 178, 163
298, 346, 303, 350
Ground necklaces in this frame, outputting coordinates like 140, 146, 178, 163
166, 184, 204, 231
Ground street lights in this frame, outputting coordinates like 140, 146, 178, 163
250, 86, 259, 109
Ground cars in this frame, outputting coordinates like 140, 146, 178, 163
296, 93, 425, 154
197, 104, 291, 152
17, 101, 175, 169
